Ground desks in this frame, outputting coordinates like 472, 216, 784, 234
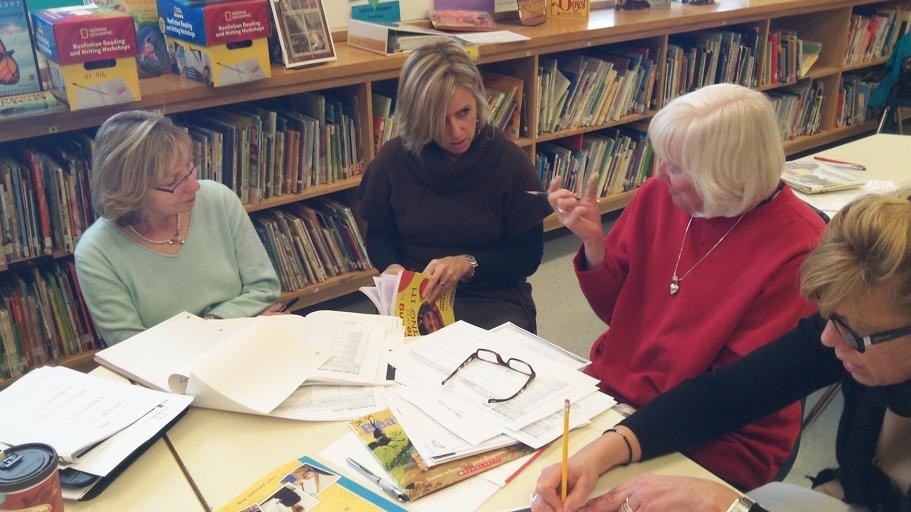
780, 132, 911, 219
0, 334, 757, 512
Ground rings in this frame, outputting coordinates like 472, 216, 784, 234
620, 498, 633, 511
439, 279, 447, 288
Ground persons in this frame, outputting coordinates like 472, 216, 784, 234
73, 106, 291, 349
545, 79, 829, 495
528, 182, 911, 511
356, 40, 546, 335
274, 486, 305, 512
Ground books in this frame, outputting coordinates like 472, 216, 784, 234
93, 312, 331, 416
833, 1, 911, 128
0, 128, 106, 384
536, 17, 824, 200
188, 88, 378, 296
357, 268, 461, 335
369, 67, 527, 160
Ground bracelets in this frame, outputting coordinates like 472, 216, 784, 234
602, 427, 633, 468
460, 252, 479, 284
725, 493, 755, 511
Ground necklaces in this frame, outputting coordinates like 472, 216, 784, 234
669, 186, 750, 299
121, 207, 187, 246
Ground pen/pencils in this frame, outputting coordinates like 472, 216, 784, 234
524, 190, 583, 200
814, 156, 864, 168
346, 456, 407, 504
561, 397, 571, 504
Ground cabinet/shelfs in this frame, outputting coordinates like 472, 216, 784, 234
1, 1, 911, 391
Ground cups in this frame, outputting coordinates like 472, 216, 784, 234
1, 442, 64, 511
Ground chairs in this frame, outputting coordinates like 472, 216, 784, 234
773, 198, 841, 483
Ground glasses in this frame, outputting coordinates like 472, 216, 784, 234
814, 294, 910, 352
442, 347, 535, 402
154, 157, 199, 194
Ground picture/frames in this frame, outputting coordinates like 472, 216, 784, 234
269, 1, 337, 68
